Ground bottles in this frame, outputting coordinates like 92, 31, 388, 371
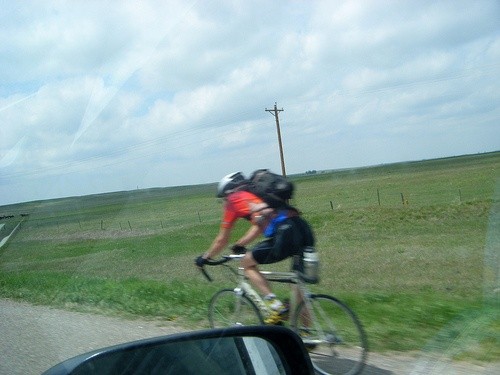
302, 246, 319, 278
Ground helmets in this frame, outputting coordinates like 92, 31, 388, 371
218, 170, 245, 195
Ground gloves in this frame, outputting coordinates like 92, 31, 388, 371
232, 245, 246, 254
195, 256, 207, 266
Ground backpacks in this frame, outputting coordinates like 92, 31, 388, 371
241, 168, 295, 200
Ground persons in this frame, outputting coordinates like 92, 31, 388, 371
193, 170, 318, 353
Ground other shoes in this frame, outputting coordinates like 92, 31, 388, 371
297, 327, 319, 339
262, 299, 287, 323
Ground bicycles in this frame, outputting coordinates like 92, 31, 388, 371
197, 245, 369, 375
